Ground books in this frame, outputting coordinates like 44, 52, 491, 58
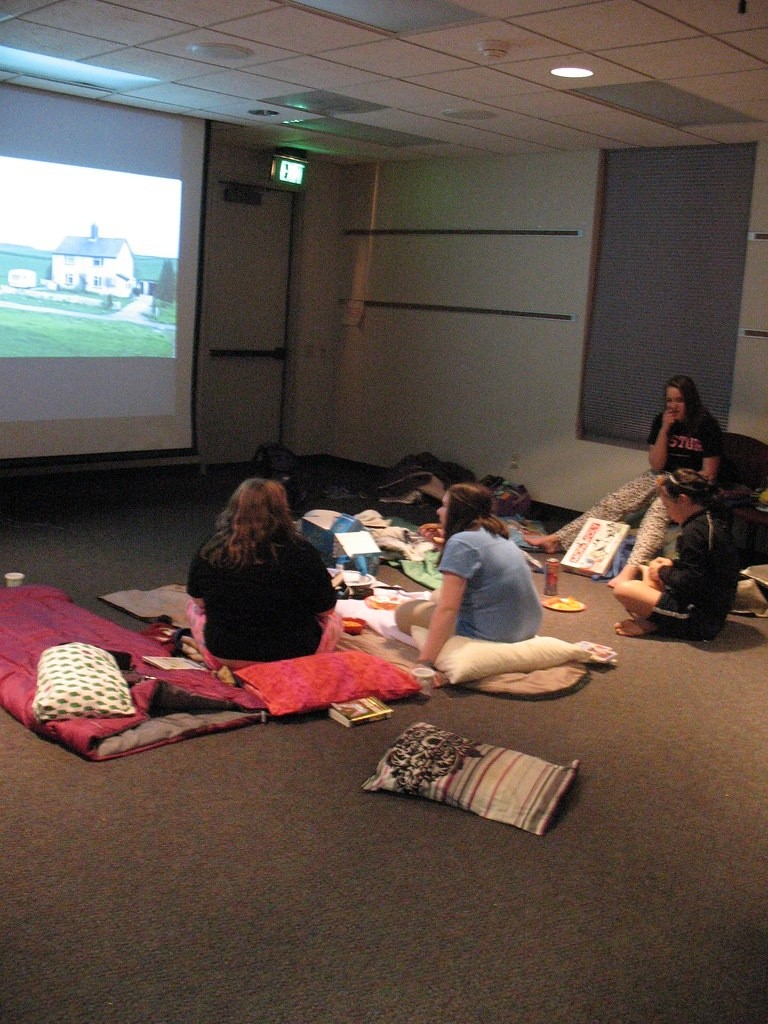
329, 695, 393, 728
141, 656, 207, 670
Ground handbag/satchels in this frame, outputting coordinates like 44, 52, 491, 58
592, 535, 665, 584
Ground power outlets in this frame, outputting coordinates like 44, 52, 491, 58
510, 451, 519, 469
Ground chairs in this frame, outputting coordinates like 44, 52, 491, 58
716, 432, 768, 544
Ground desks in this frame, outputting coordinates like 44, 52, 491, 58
725, 505, 768, 560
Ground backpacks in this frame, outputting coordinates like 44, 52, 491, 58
478, 474, 532, 517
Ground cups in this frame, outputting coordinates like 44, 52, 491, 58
412, 667, 435, 692
4, 573, 25, 587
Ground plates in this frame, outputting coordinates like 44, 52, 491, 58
540, 597, 587, 612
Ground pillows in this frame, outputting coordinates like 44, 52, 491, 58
362, 721, 581, 837
410, 625, 594, 686
233, 649, 423, 716
31, 641, 138, 725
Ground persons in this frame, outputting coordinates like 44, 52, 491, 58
186, 478, 342, 669
525, 377, 720, 588
614, 470, 739, 641
395, 483, 542, 688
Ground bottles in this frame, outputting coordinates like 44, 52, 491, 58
335, 555, 350, 570
508, 452, 522, 487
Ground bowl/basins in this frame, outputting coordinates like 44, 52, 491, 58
339, 570, 376, 591
340, 617, 366, 635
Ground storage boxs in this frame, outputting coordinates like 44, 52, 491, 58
332, 514, 364, 534
561, 517, 631, 578
316, 551, 379, 577
302, 516, 383, 559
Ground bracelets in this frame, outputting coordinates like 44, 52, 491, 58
415, 658, 433, 670
